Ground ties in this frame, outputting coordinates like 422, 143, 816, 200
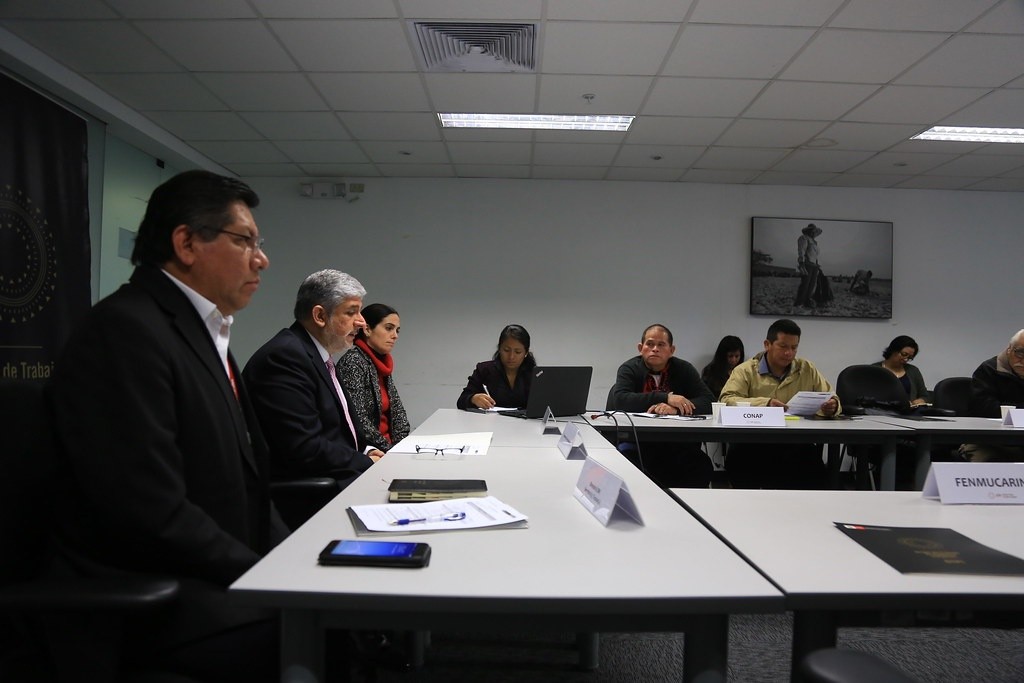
325, 355, 358, 454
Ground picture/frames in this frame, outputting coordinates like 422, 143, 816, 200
751, 216, 894, 319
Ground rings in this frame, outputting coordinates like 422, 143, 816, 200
683, 403, 686, 407
654, 404, 657, 407
659, 408, 664, 412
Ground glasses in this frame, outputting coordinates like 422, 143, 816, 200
1012, 346, 1024, 357
193, 222, 265, 256
900, 352, 914, 362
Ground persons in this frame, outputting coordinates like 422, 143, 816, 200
613, 323, 714, 489
239, 269, 386, 532
850, 269, 872, 293
869, 335, 933, 416
456, 324, 536, 411
32, 168, 412, 683
333, 304, 411, 456
794, 223, 823, 307
957, 329, 1024, 463
719, 319, 842, 490
701, 335, 745, 399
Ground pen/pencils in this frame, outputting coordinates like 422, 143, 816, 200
482, 384, 490, 397
679, 415, 706, 419
388, 512, 466, 526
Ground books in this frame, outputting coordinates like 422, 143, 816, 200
388, 479, 488, 503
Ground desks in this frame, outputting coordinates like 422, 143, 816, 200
224, 409, 1024, 682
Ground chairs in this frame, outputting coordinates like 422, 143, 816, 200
0, 382, 179, 682
836, 365, 919, 490
933, 377, 973, 416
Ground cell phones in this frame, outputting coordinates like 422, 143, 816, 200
319, 539, 431, 571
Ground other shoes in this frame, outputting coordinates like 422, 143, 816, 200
363, 630, 412, 672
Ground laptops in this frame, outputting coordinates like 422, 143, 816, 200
497, 366, 593, 419
